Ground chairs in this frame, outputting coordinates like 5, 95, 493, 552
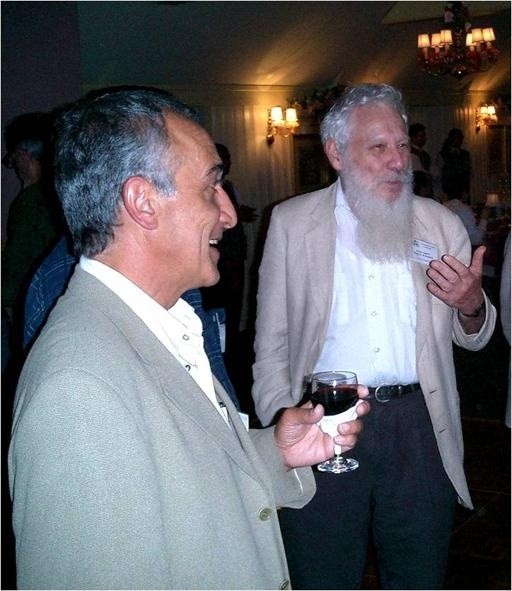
481, 234, 508, 288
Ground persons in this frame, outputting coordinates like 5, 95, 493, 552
8, 90, 370, 591
407, 123, 511, 428
251, 84, 497, 590
3, 111, 249, 412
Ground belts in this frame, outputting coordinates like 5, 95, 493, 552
369, 383, 423, 400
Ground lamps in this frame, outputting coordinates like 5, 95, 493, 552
416, 1, 499, 80
266, 106, 300, 145
476, 106, 498, 133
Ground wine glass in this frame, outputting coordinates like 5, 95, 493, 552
308, 366, 362, 475
